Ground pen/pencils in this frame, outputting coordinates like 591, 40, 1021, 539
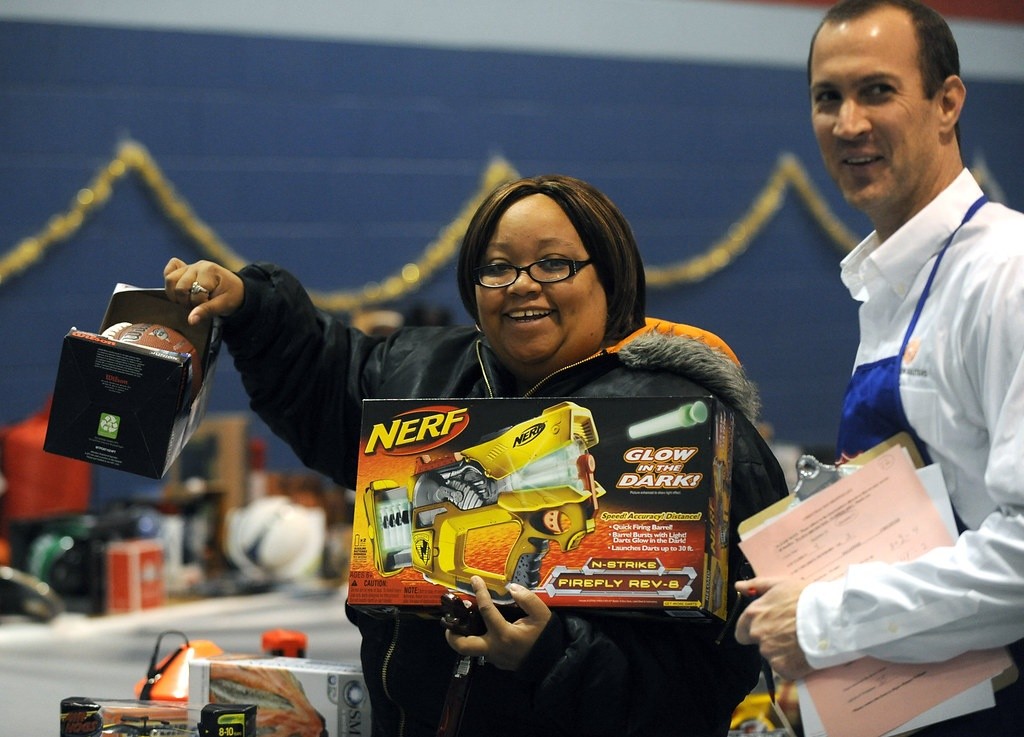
746, 587, 777, 706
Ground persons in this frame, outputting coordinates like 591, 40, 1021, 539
734, 0, 1024, 737
164, 170, 787, 736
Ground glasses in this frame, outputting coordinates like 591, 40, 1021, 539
471, 257, 597, 289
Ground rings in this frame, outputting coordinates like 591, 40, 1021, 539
191, 282, 208, 293
